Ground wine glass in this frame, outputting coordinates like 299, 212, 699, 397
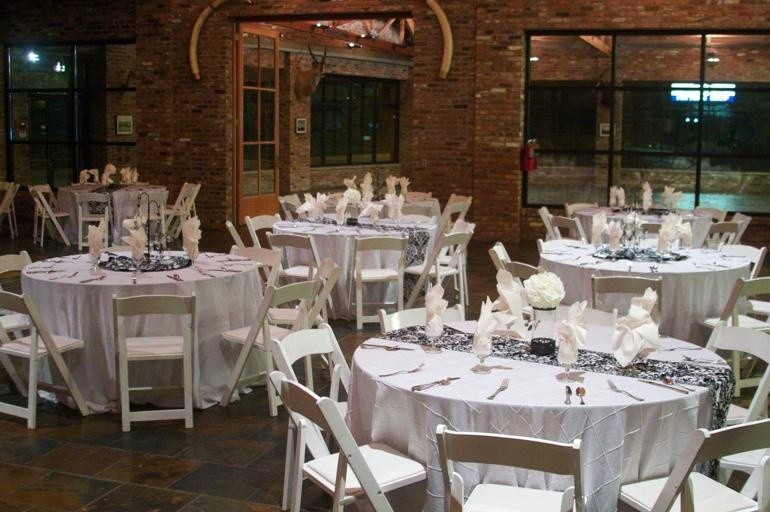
470, 336, 495, 374
629, 350, 643, 377
424, 320, 443, 354
556, 334, 579, 382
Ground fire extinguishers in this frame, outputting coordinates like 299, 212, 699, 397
521, 139, 535, 172
532, 139, 539, 169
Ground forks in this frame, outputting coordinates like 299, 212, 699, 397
607, 379, 644, 401
487, 377, 509, 400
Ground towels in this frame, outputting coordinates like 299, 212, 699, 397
633, 286, 657, 317
600, 182, 700, 236
304, 171, 410, 218
496, 270, 529, 319
122, 218, 147, 234
423, 284, 449, 338
474, 297, 514, 359
181, 214, 204, 259
554, 300, 589, 365
611, 308, 658, 368
123, 226, 148, 261
79, 164, 140, 185
84, 225, 105, 261
601, 237, 693, 252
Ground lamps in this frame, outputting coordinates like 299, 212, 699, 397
705, 50, 720, 64
316, 22, 378, 48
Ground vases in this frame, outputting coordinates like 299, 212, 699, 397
529, 306, 558, 356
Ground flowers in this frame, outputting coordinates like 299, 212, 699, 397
524, 272, 566, 308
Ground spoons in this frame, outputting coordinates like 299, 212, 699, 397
576, 386, 586, 405
379, 362, 426, 377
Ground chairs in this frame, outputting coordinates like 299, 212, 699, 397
376, 305, 466, 335
536, 235, 587, 261
266, 231, 338, 322
698, 237, 726, 249
244, 214, 282, 288
228, 245, 282, 297
716, 426, 770, 498
268, 257, 342, 335
2, 182, 20, 241
74, 192, 115, 250
717, 241, 768, 284
436, 217, 475, 306
28, 183, 60, 239
443, 194, 474, 219
156, 182, 196, 243
278, 194, 306, 221
435, 422, 588, 512
692, 207, 753, 239
30, 187, 71, 248
1, 250, 33, 340
267, 323, 352, 511
220, 274, 323, 416
704, 319, 769, 425
268, 371, 425, 512
501, 259, 541, 285
226, 220, 245, 248
620, 416, 770, 512
696, 274, 769, 397
345, 234, 409, 329
0, 291, 90, 430
537, 201, 600, 238
112, 292, 197, 432
436, 200, 472, 239
589, 273, 662, 335
490, 240, 511, 274
167, 184, 201, 239
404, 231, 471, 309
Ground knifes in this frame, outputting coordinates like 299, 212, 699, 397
640, 377, 690, 394
564, 385, 573, 404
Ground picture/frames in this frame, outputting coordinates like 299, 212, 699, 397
115, 114, 134, 136
295, 118, 307, 135
599, 121, 617, 139
